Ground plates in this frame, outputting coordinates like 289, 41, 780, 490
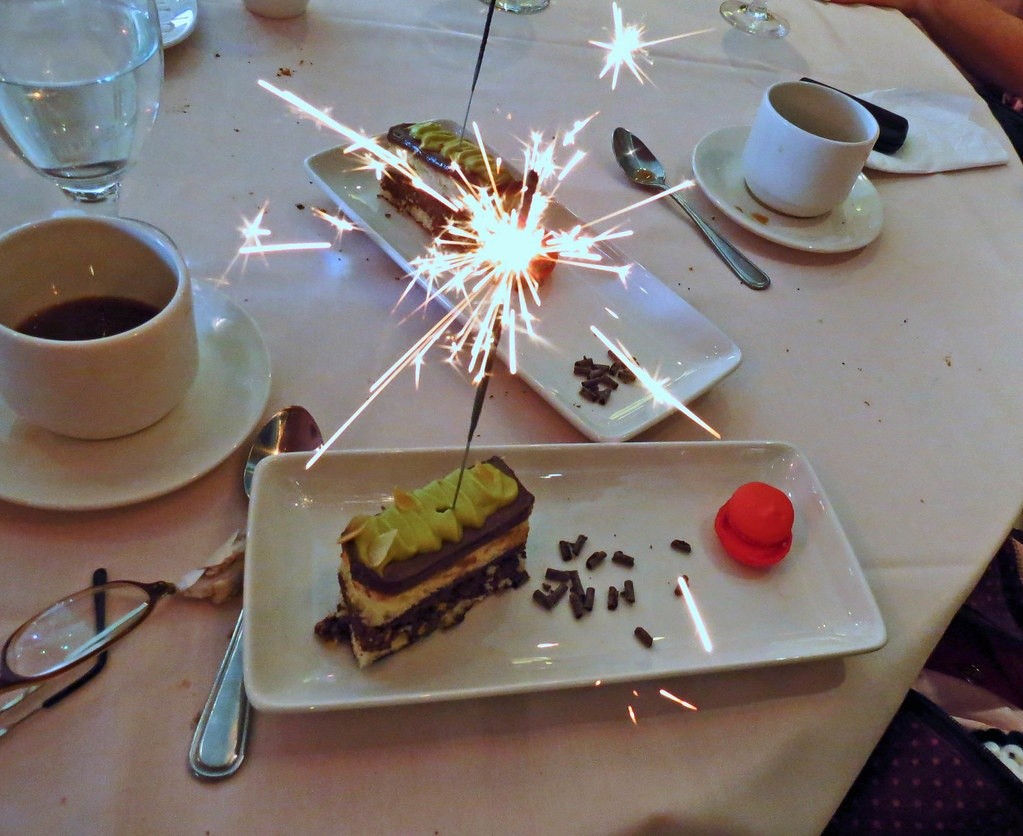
0, 278, 271, 511
242, 440, 889, 714
692, 125, 883, 252
303, 119, 743, 442
155, 0, 197, 49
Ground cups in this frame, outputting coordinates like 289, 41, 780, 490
742, 82, 880, 217
245, 0, 308, 19
0, 215, 199, 440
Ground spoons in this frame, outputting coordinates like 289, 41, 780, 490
188, 405, 324, 781
612, 126, 771, 290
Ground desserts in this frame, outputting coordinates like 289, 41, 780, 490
714, 483, 794, 569
314, 455, 536, 669
377, 120, 520, 250
502, 218, 559, 293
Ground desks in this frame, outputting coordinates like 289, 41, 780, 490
0, 1, 1023, 836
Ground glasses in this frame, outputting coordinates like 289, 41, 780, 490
1, 569, 177, 734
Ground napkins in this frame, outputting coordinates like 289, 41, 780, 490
855, 88, 1010, 175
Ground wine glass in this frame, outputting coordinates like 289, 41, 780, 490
479, 0, 550, 14
720, 0, 789, 38
0, 0, 165, 215
